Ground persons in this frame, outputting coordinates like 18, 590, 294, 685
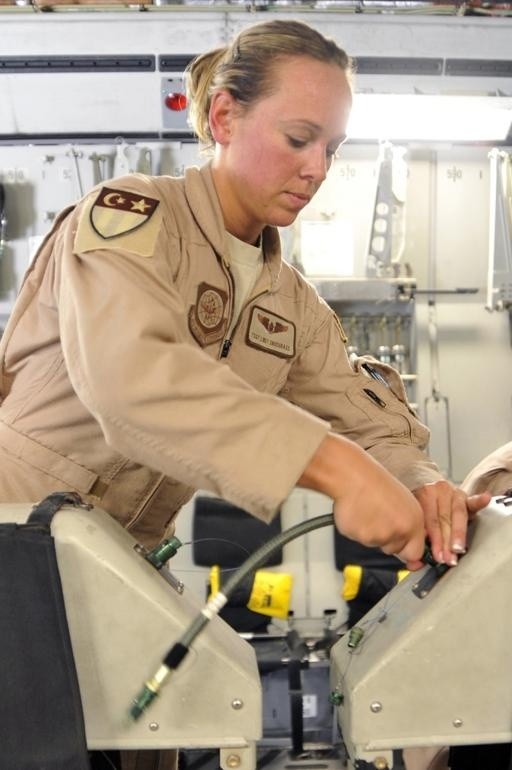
1, 20, 493, 573
398, 434, 512, 770
